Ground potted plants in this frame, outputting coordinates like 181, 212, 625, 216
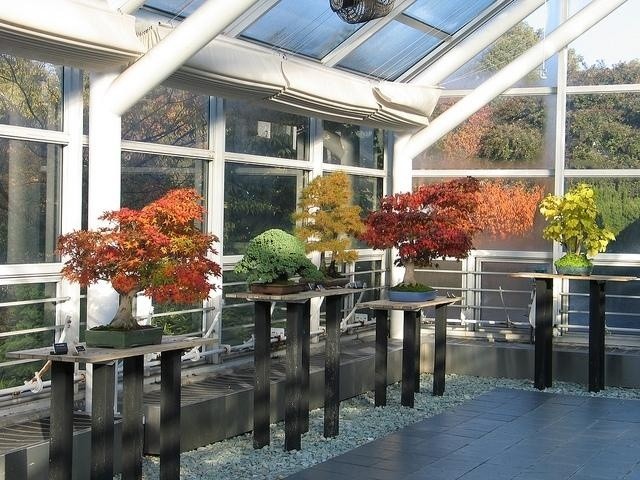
355, 174, 481, 303
51, 183, 222, 350
290, 169, 368, 289
537, 182, 617, 276
232, 227, 325, 297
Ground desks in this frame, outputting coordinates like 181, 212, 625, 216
507, 271, 637, 393
355, 295, 466, 409
225, 286, 368, 453
5, 335, 221, 479
